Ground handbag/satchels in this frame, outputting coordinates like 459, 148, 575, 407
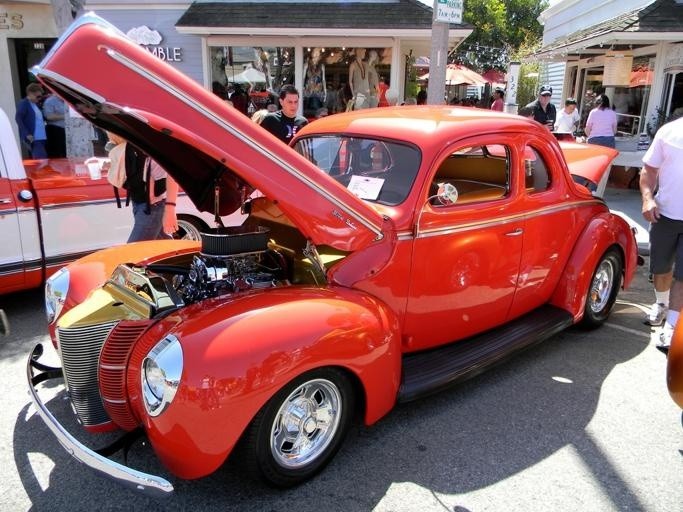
106, 142, 131, 208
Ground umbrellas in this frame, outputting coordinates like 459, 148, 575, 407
417, 59, 490, 105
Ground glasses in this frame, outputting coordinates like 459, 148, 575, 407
35, 95, 42, 98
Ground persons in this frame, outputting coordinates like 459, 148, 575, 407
638, 116, 683, 350
518, 106, 535, 120
490, 89, 504, 112
525, 84, 556, 132
44, 92, 65, 158
212, 79, 310, 147
106, 132, 179, 243
584, 94, 618, 149
553, 98, 580, 136
303, 48, 380, 110
14, 83, 49, 160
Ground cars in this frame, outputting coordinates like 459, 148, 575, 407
25, 12, 640, 504
250, 85, 270, 96
0, 98, 265, 345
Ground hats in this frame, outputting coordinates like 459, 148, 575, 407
539, 86, 552, 96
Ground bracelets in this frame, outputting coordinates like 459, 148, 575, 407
164, 202, 176, 206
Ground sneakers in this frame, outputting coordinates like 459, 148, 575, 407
641, 303, 669, 326
656, 328, 674, 349
